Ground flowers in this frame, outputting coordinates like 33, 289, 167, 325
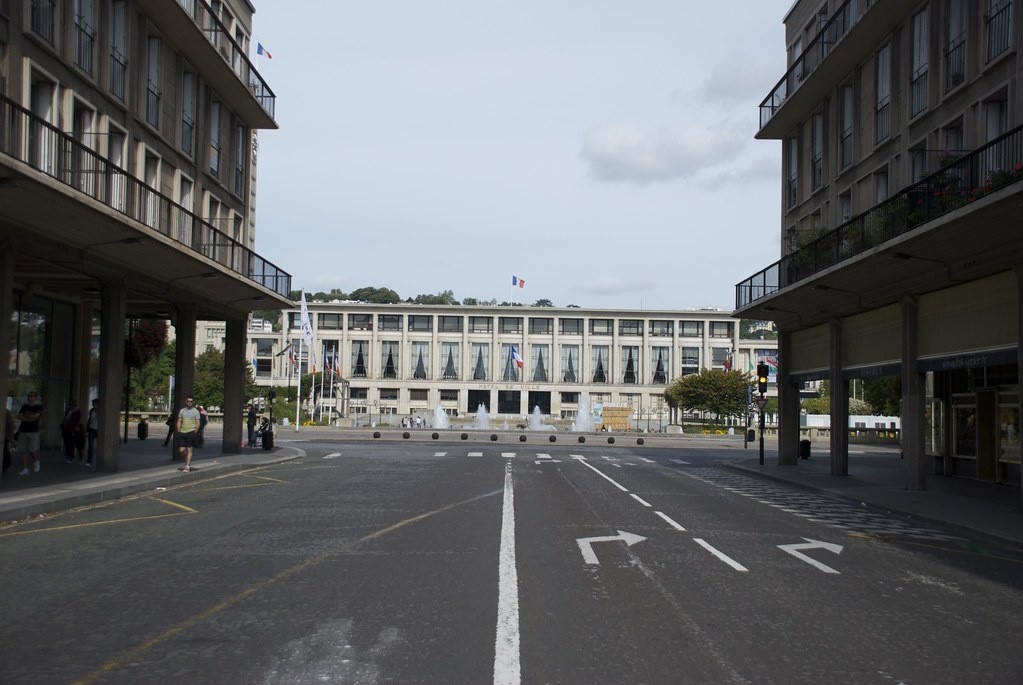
933, 149, 1023, 209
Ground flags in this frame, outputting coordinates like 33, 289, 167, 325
252, 346, 340, 376
302, 288, 313, 346
511, 345, 524, 368
723, 353, 779, 371
512, 276, 525, 289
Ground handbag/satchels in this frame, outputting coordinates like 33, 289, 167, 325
14, 432, 18, 440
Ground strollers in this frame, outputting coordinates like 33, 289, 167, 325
252, 418, 269, 448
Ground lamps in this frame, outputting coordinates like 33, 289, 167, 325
890, 252, 950, 279
760, 306, 801, 326
172, 273, 218, 284
811, 284, 861, 307
226, 296, 267, 306
86, 236, 147, 252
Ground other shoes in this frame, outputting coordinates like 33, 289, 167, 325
34, 460, 40, 472
18, 468, 30, 475
183, 465, 190, 472
66, 458, 73, 464
86, 462, 93, 467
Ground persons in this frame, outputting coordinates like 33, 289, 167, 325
60, 398, 87, 465
87, 398, 99, 467
246, 403, 269, 445
17, 392, 44, 475
176, 396, 208, 472
401, 416, 425, 429
3, 409, 16, 472
162, 408, 174, 446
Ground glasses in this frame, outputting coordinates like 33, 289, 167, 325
188, 401, 193, 402
27, 395, 34, 397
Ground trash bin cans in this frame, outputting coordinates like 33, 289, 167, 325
748, 429, 755, 442
138, 417, 149, 439
262, 430, 274, 450
799, 440, 811, 459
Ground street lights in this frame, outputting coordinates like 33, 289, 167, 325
268, 388, 276, 431
757, 361, 769, 465
